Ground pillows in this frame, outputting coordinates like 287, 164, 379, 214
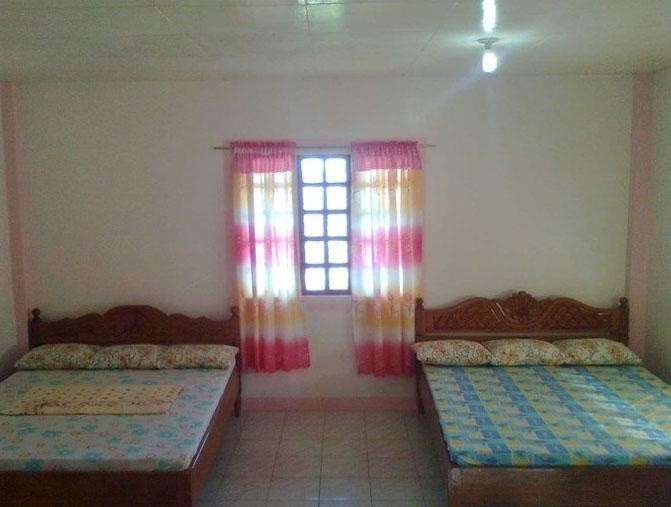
410, 333, 643, 365
5, 341, 239, 369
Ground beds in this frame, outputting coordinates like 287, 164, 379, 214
411, 285, 669, 505
0, 304, 241, 505
